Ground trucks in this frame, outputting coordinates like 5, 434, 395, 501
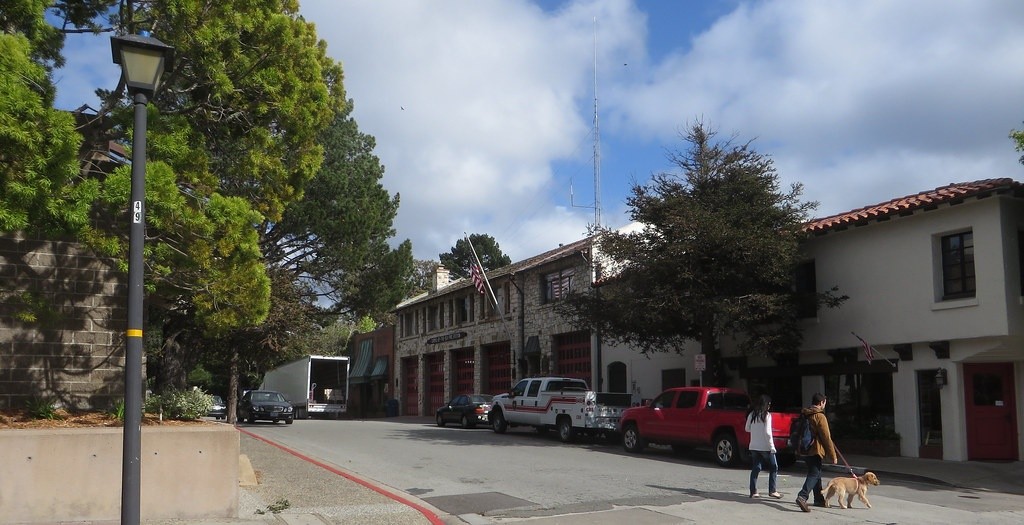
257, 355, 350, 418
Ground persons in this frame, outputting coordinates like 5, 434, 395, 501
796, 392, 838, 513
745, 395, 784, 499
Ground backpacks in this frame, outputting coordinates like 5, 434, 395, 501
790, 412, 824, 453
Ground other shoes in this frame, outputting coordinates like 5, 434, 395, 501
796, 496, 811, 512
814, 499, 825, 507
769, 492, 784, 499
749, 493, 761, 499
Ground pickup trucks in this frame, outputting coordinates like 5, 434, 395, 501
488, 377, 634, 443
617, 386, 806, 466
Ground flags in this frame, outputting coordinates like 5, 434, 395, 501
859, 339, 875, 365
468, 264, 485, 294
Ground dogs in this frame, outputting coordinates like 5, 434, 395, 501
821, 472, 879, 509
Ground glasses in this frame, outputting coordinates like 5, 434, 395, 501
768, 403, 773, 407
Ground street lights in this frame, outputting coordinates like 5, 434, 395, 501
110, 31, 178, 525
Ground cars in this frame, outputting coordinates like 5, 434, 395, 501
237, 390, 295, 424
435, 394, 495, 429
199, 395, 227, 420
